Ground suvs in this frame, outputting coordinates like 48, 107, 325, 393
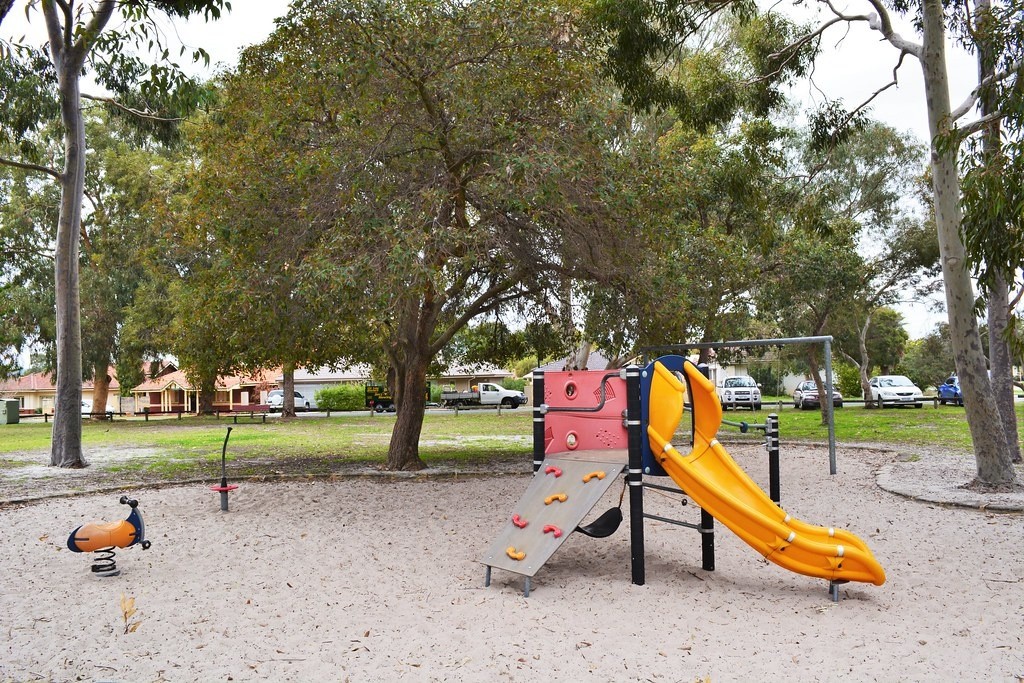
716, 375, 763, 412
266, 389, 310, 414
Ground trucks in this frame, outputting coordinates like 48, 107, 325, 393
365, 380, 431, 413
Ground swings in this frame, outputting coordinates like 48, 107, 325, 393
750, 345, 782, 427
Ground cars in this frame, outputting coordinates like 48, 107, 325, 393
862, 375, 924, 409
937, 375, 963, 407
792, 380, 843, 410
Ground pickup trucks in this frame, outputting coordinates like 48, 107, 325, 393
440, 382, 529, 410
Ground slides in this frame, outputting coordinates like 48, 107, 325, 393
645, 354, 885, 586
